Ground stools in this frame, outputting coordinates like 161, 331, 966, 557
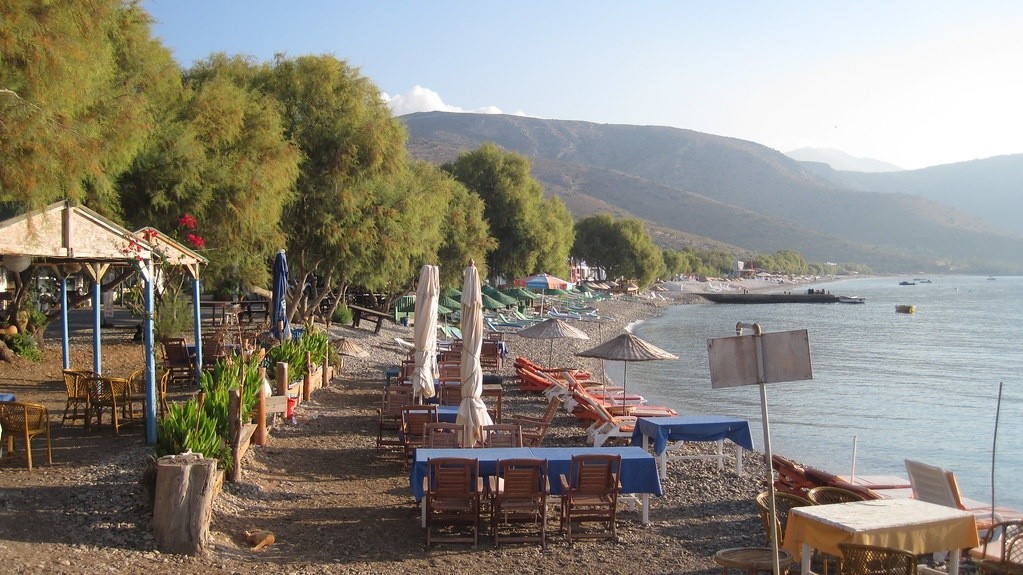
225, 313, 234, 325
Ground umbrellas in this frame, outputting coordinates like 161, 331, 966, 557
622, 283, 638, 288
431, 264, 440, 379
481, 285, 538, 323
517, 317, 590, 381
585, 280, 619, 289
272, 250, 291, 341
514, 273, 568, 318
573, 331, 679, 416
400, 286, 506, 341
539, 284, 595, 310
333, 335, 370, 366
455, 257, 494, 448
649, 285, 668, 295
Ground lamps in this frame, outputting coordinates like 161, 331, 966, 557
2, 255, 32, 272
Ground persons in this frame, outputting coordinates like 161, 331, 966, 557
409, 265, 436, 405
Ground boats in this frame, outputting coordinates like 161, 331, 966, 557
838, 294, 867, 303
896, 302, 916, 314
899, 278, 933, 286
986, 276, 997, 281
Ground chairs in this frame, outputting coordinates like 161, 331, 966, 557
441, 291, 614, 344
0, 331, 255, 472
374, 332, 1023, 575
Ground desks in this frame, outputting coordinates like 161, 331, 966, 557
403, 405, 461, 463
411, 448, 541, 528
716, 546, 792, 575
402, 376, 461, 403
181, 343, 242, 362
484, 341, 508, 367
632, 415, 753, 477
231, 300, 270, 323
782, 498, 980, 575
482, 384, 502, 423
187, 302, 227, 326
530, 447, 662, 524
348, 305, 394, 333
